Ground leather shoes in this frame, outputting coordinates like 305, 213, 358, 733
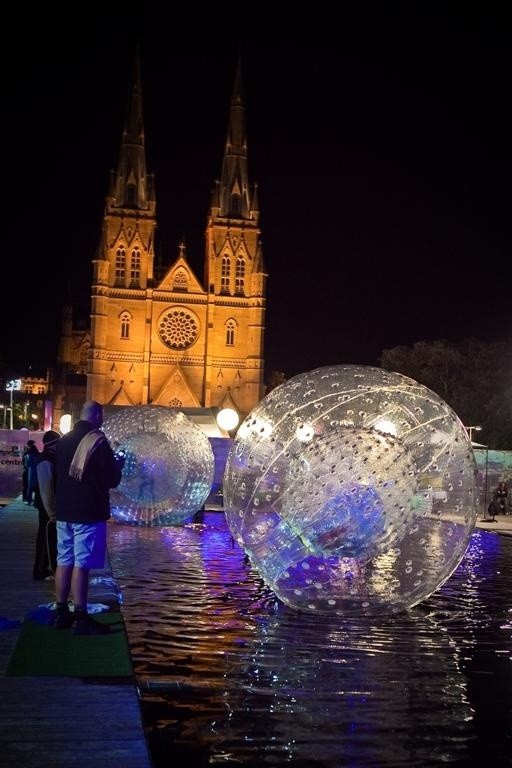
55, 615, 110, 635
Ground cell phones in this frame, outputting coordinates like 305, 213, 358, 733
116, 450, 125, 461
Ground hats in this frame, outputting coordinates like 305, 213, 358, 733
43, 431, 61, 446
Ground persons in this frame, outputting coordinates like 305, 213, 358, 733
125, 463, 156, 498
495, 481, 511, 516
22, 431, 62, 581
54, 399, 121, 633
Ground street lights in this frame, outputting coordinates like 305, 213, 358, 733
0, 404, 13, 430
461, 424, 483, 451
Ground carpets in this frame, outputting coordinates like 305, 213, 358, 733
4, 612, 137, 680
